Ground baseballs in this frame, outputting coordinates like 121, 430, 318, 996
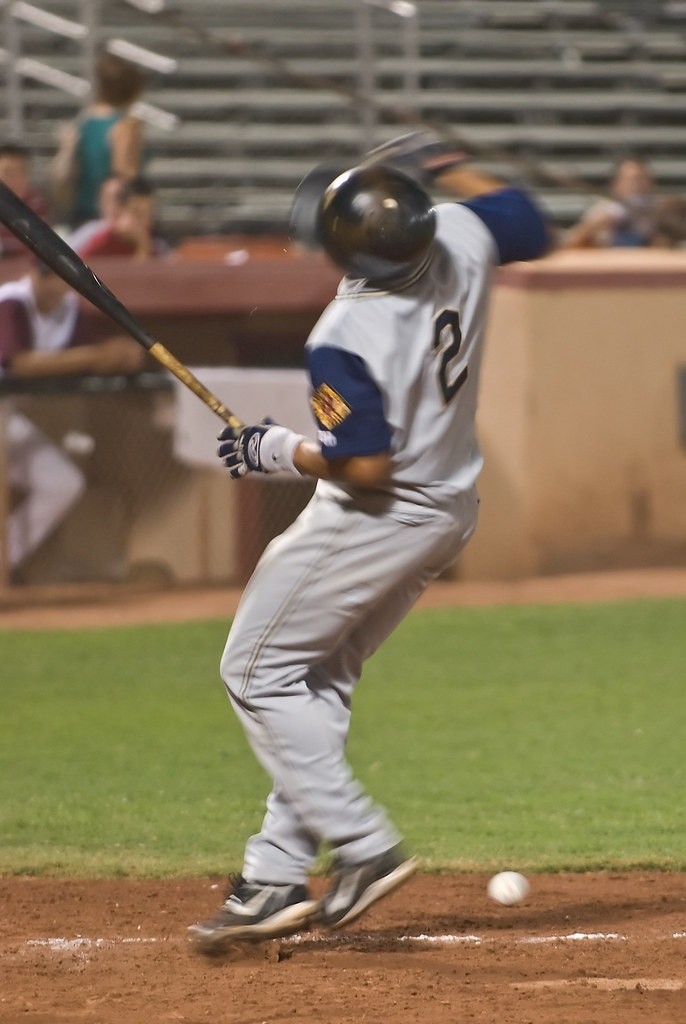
486, 871, 530, 906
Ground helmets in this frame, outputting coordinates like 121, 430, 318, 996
316, 165, 437, 289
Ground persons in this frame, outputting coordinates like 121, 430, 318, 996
191, 148, 553, 952
0, 47, 165, 582
560, 152, 686, 247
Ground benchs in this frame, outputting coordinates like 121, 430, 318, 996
0, 0, 686, 237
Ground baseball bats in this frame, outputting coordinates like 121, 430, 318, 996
0, 180, 247, 429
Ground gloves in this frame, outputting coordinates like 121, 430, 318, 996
217, 416, 309, 480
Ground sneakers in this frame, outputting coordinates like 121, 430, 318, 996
323, 845, 420, 929
186, 871, 320, 943
